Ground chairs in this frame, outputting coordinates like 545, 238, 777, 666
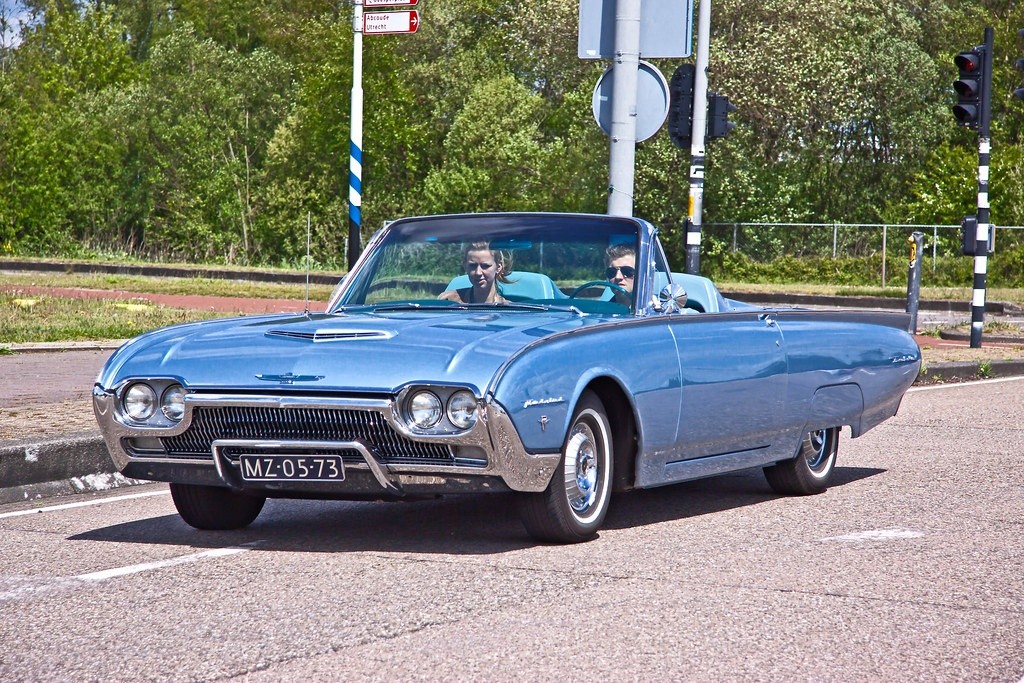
598, 272, 720, 314
444, 272, 555, 304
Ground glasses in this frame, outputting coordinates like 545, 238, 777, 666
604, 265, 635, 279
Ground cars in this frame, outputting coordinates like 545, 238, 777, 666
91, 211, 922, 543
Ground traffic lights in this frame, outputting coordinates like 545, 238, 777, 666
951, 51, 982, 125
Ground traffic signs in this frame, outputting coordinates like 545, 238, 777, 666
361, 10, 421, 35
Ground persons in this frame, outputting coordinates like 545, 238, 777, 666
437, 241, 514, 304
605, 243, 699, 313
